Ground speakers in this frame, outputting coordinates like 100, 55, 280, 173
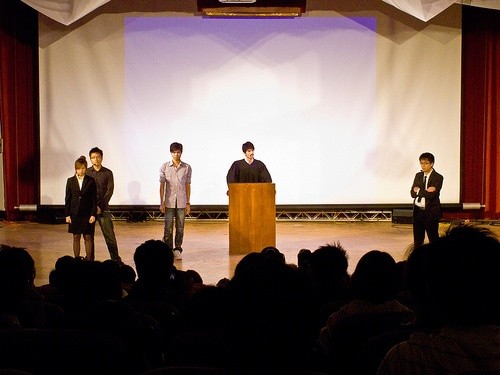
392, 209, 414, 224
36, 207, 66, 224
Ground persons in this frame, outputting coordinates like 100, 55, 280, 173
159, 142, 192, 261
82, 147, 124, 265
226, 141, 272, 194
411, 153, 446, 257
1, 222, 500, 375
65, 155, 97, 263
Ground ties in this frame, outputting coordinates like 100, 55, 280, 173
417, 176, 427, 203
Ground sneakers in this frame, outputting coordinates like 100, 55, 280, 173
175, 250, 182, 259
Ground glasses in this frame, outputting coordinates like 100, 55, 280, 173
420, 162, 431, 165
